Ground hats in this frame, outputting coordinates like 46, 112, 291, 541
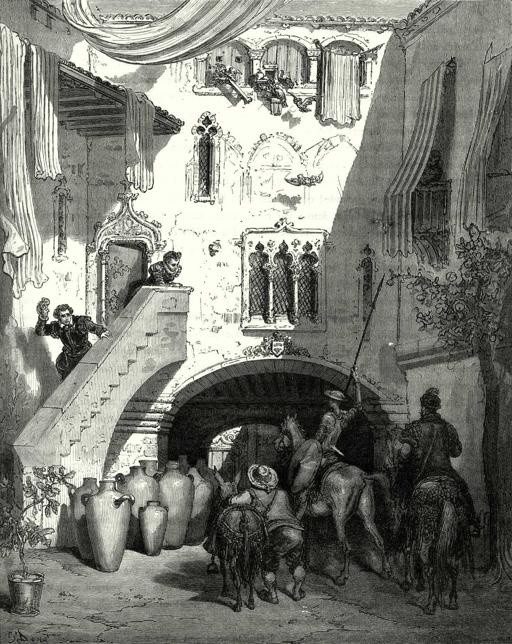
247, 463, 281, 494
324, 388, 347, 405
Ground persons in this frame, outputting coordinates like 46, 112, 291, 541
284, 368, 363, 524
226, 464, 305, 603
393, 391, 463, 500
32, 295, 111, 383
124, 250, 195, 308
